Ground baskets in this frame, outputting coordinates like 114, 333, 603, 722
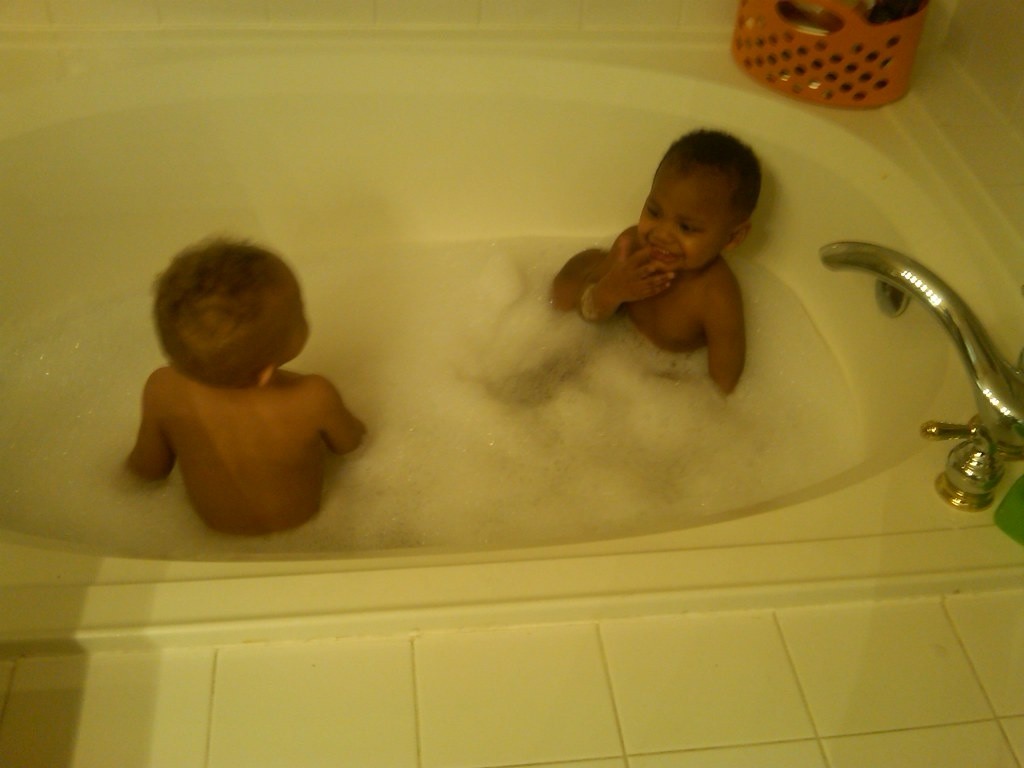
729, 0, 930, 110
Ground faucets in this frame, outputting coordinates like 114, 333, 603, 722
819, 240, 1024, 514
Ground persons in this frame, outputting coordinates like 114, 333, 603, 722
123, 238, 367, 536
550, 129, 763, 397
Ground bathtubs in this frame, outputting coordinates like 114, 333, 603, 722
0, 1, 1023, 768
